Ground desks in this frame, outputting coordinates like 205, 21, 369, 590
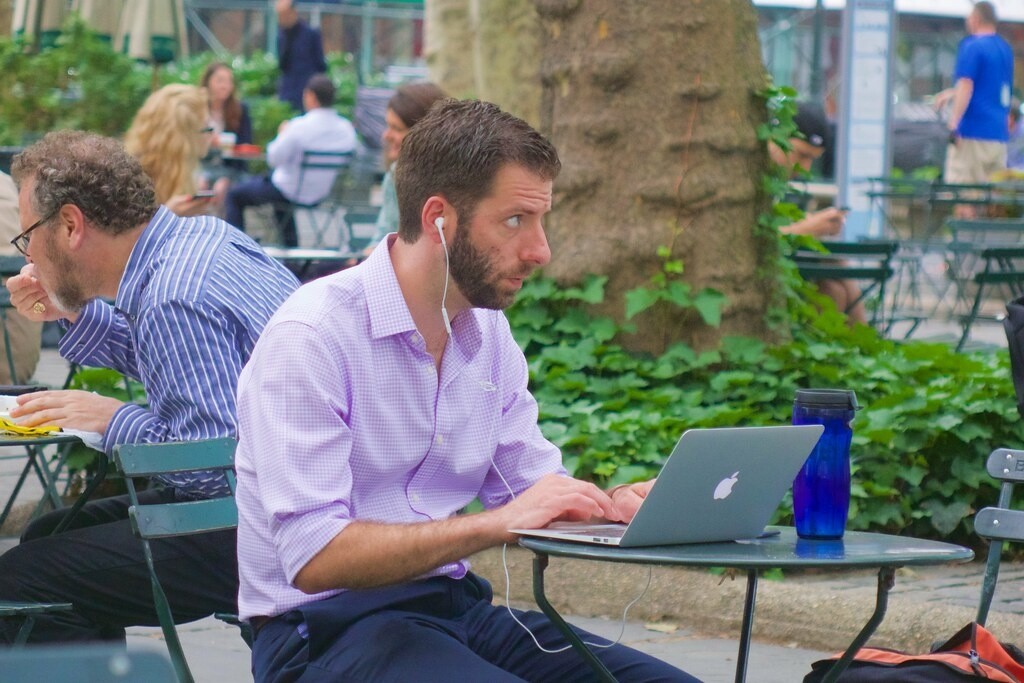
522, 527, 974, 682
0, 432, 117, 543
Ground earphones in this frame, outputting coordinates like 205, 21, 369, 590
435, 217, 447, 244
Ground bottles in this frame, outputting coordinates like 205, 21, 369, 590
793, 389, 864, 539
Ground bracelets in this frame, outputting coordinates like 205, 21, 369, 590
606, 484, 628, 498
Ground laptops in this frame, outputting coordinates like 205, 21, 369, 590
507, 424, 824, 548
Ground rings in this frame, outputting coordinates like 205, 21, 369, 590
33, 301, 46, 313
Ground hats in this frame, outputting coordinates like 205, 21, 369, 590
385, 80, 450, 133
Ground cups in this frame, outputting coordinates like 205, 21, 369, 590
218, 132, 236, 159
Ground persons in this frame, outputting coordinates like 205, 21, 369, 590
1005, 95, 1024, 172
225, 76, 359, 249
276, 0, 328, 113
122, 82, 228, 219
201, 62, 251, 202
348, 81, 451, 271
931, 0, 1015, 269
234, 98, 704, 683
0, 170, 45, 386
0, 127, 300, 652
765, 100, 867, 327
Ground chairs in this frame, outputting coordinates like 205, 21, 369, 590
111, 439, 244, 683
0, 254, 80, 642
973, 449, 1024, 629
254, 152, 380, 273
760, 175, 1023, 353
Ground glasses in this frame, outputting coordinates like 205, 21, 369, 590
11, 201, 78, 257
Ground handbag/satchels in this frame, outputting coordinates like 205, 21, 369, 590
803, 621, 1024, 683
